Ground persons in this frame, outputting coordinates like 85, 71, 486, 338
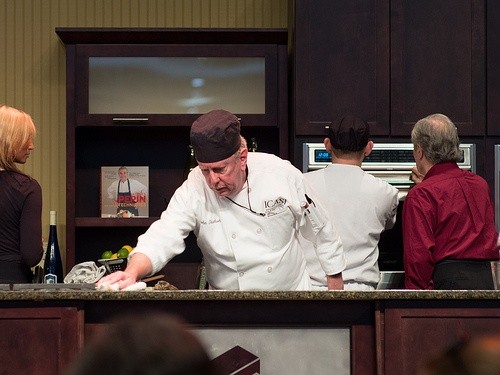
0, 106, 44, 284
95, 110, 349, 292
440, 326, 500, 375
65, 316, 217, 375
107, 167, 146, 216
303, 115, 398, 291
402, 114, 500, 290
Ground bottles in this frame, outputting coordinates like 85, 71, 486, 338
34, 266, 44, 283
43, 210, 63, 283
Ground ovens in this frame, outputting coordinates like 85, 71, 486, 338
304, 144, 477, 290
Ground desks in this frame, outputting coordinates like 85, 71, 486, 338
1, 288, 500, 375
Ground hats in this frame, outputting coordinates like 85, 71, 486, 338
190, 109, 240, 163
328, 107, 370, 152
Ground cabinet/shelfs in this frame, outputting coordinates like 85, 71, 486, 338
55, 25, 289, 278
287, 0, 500, 141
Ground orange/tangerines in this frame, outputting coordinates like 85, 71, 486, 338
102, 245, 135, 259
